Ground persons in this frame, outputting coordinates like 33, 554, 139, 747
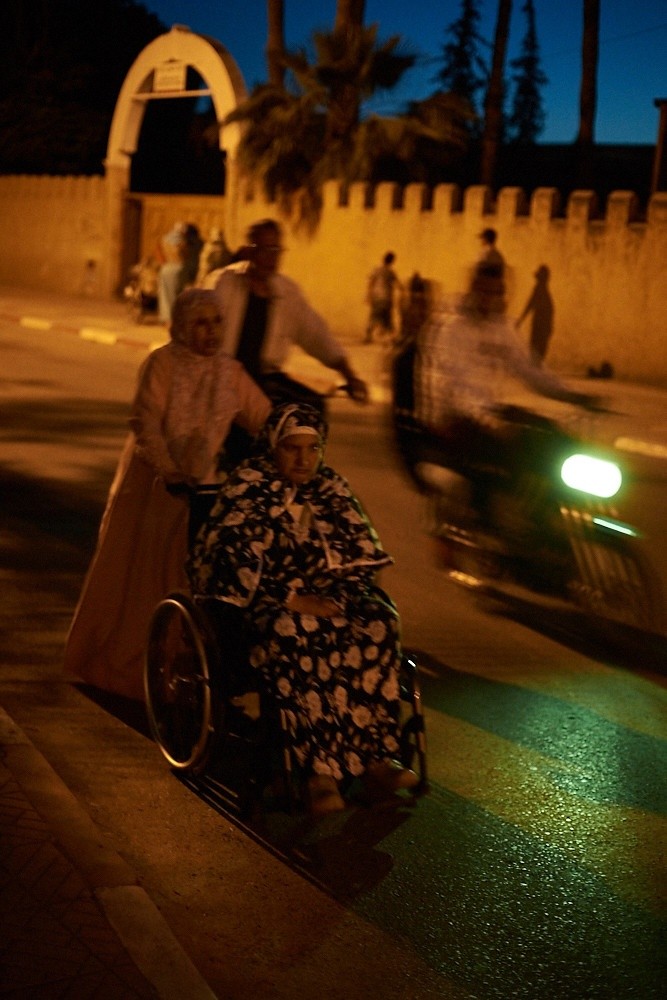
192, 404, 419, 809
124, 220, 610, 561
61, 290, 272, 706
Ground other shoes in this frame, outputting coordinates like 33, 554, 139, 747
367, 757, 419, 788
308, 774, 345, 810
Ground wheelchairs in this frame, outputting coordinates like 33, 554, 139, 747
143, 479, 430, 798
